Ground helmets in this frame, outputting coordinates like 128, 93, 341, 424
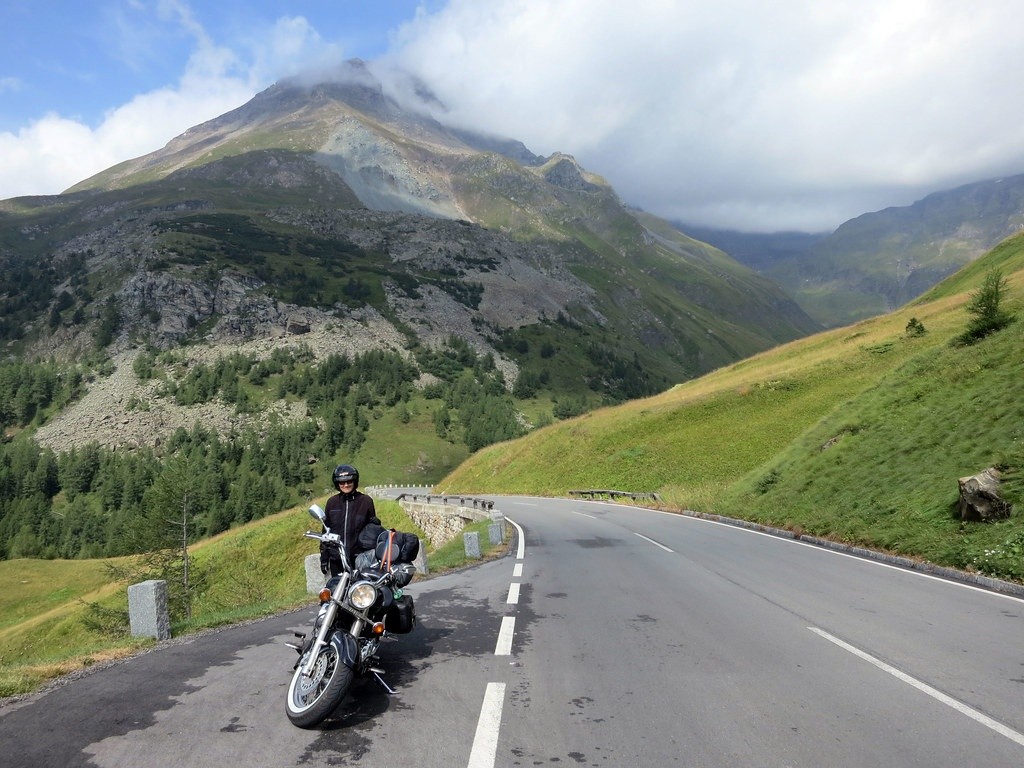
332, 465, 359, 490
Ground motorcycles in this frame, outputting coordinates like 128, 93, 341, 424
283, 504, 403, 731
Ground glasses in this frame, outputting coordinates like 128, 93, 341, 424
338, 480, 353, 485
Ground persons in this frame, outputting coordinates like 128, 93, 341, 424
320, 463, 377, 574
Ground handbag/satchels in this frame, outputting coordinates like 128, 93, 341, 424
354, 530, 419, 634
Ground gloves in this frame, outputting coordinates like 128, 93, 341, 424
369, 516, 382, 525
320, 560, 329, 575
